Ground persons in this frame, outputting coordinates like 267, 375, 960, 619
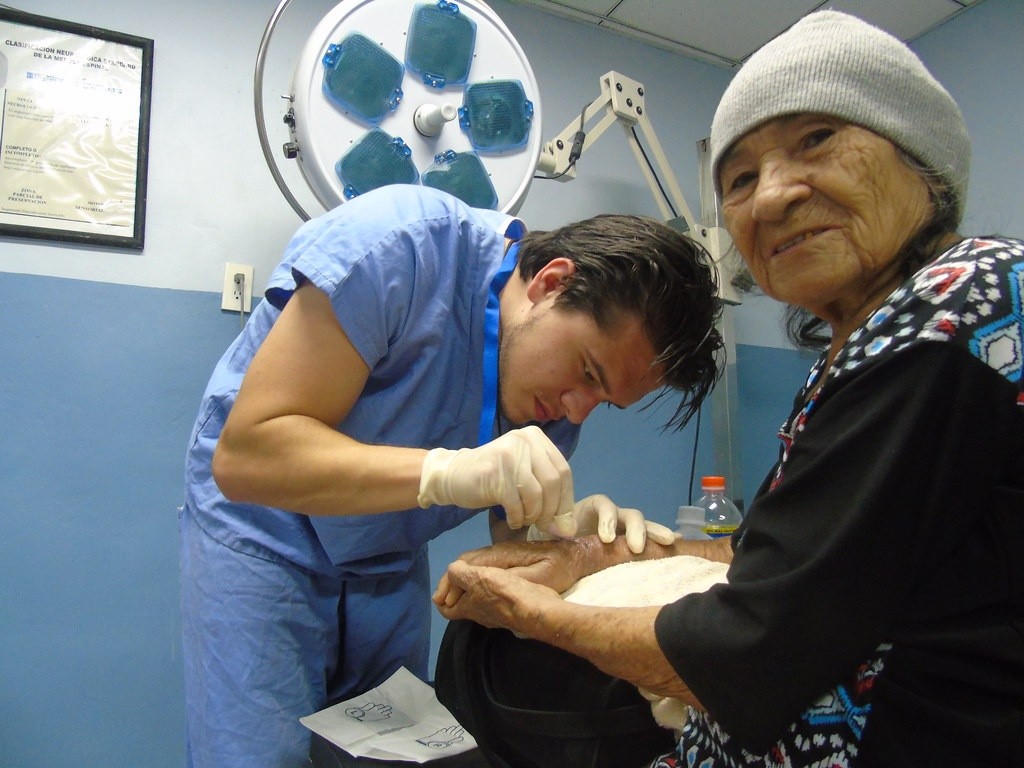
178, 183, 726, 768
433, 10, 1024, 768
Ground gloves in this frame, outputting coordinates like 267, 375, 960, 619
418, 425, 578, 539
526, 494, 683, 554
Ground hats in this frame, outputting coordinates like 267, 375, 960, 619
711, 9, 968, 226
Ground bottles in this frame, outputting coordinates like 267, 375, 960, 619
670, 475, 745, 541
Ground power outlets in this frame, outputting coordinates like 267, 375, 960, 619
221, 262, 254, 313
